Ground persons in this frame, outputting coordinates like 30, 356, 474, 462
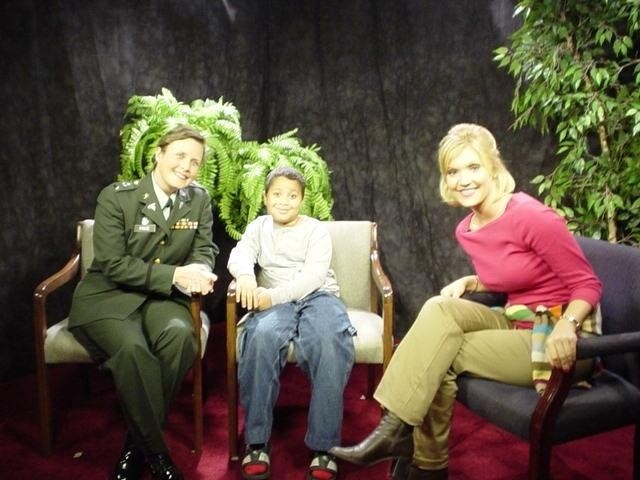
225, 163, 356, 480
324, 121, 603, 480
65, 122, 221, 480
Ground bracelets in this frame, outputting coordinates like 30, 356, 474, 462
559, 314, 582, 331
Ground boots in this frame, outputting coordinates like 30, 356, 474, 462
328, 408, 414, 479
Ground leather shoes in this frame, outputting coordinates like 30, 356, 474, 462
114, 447, 142, 479
145, 451, 183, 479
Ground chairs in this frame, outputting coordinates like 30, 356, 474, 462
224, 220, 394, 462
452, 236, 640, 479
34, 213, 205, 450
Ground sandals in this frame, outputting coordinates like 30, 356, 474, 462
240, 444, 271, 480
306, 447, 338, 480
